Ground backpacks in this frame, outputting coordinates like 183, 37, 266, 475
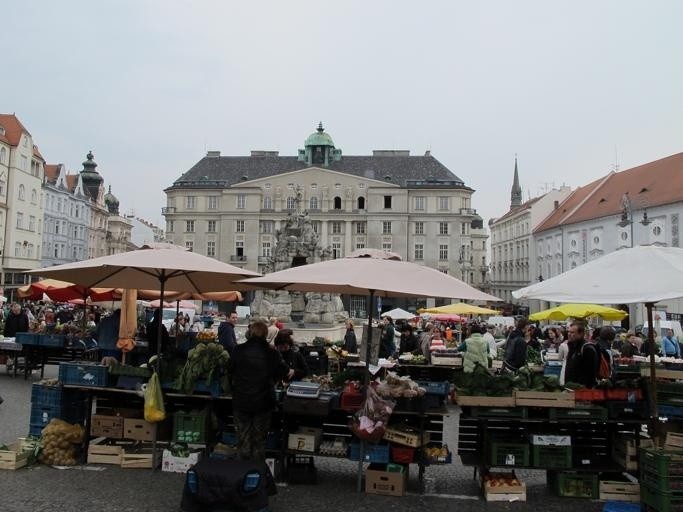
581, 342, 609, 378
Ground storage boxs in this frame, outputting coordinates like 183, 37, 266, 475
665, 432, 682, 447
431, 338, 444, 346
91, 414, 123, 439
612, 437, 654, 472
431, 352, 462, 365
123, 418, 157, 441
599, 482, 641, 504
479, 467, 527, 502
638, 367, 683, 379
265, 457, 281, 479
179, 331, 216, 352
287, 426, 323, 452
491, 359, 540, 370
365, 462, 406, 497
162, 448, 202, 474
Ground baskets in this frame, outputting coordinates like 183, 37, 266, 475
174, 410, 211, 443
533, 443, 572, 471
636, 444, 683, 512
58, 362, 109, 387
29, 381, 61, 439
285, 455, 318, 485
15, 333, 66, 347
348, 427, 429, 464
558, 471, 598, 499
492, 442, 530, 467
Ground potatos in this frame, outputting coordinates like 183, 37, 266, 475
196, 332, 218, 340
41, 378, 58, 385
425, 447, 448, 459
36, 419, 85, 467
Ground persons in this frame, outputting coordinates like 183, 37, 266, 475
266, 318, 280, 347
419, 320, 514, 344
378, 315, 395, 359
343, 319, 357, 354
527, 321, 683, 391
171, 309, 238, 347
399, 325, 420, 364
275, 329, 314, 387
0, 300, 111, 337
228, 321, 285, 455
501, 317, 530, 377
456, 324, 491, 373
481, 327, 498, 373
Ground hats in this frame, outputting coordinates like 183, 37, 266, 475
275, 329, 293, 345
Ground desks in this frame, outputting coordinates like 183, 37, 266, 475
0, 336, 23, 377
21, 343, 97, 381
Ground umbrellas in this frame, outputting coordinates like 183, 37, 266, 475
380, 308, 416, 320
20, 248, 265, 472
233, 247, 505, 490
427, 314, 466, 324
414, 302, 501, 346
113, 290, 245, 336
528, 303, 628, 321
511, 245, 683, 452
15, 277, 116, 341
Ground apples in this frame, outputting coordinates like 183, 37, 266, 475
413, 354, 426, 361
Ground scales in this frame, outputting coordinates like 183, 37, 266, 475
286, 381, 321, 398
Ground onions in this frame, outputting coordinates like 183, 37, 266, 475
491, 478, 520, 487
